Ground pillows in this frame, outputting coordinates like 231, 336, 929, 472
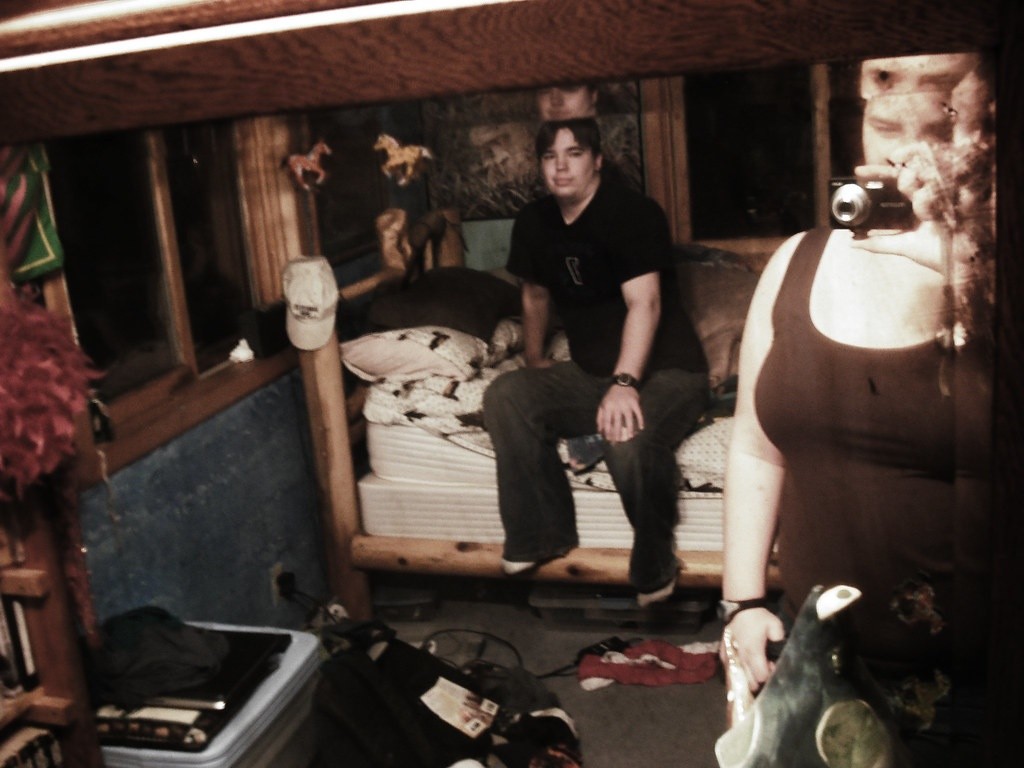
337, 327, 487, 380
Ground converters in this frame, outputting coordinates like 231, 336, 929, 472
576, 635, 623, 664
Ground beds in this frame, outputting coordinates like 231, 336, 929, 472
296, 229, 765, 587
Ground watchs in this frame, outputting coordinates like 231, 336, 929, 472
604, 372, 641, 392
715, 597, 767, 622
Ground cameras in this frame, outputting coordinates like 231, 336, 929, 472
827, 176, 912, 230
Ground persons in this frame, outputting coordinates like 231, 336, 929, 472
482, 116, 712, 607
720, 52, 977, 696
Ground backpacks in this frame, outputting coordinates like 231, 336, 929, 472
313, 614, 585, 768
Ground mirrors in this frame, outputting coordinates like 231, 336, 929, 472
0, 0, 1024, 768
